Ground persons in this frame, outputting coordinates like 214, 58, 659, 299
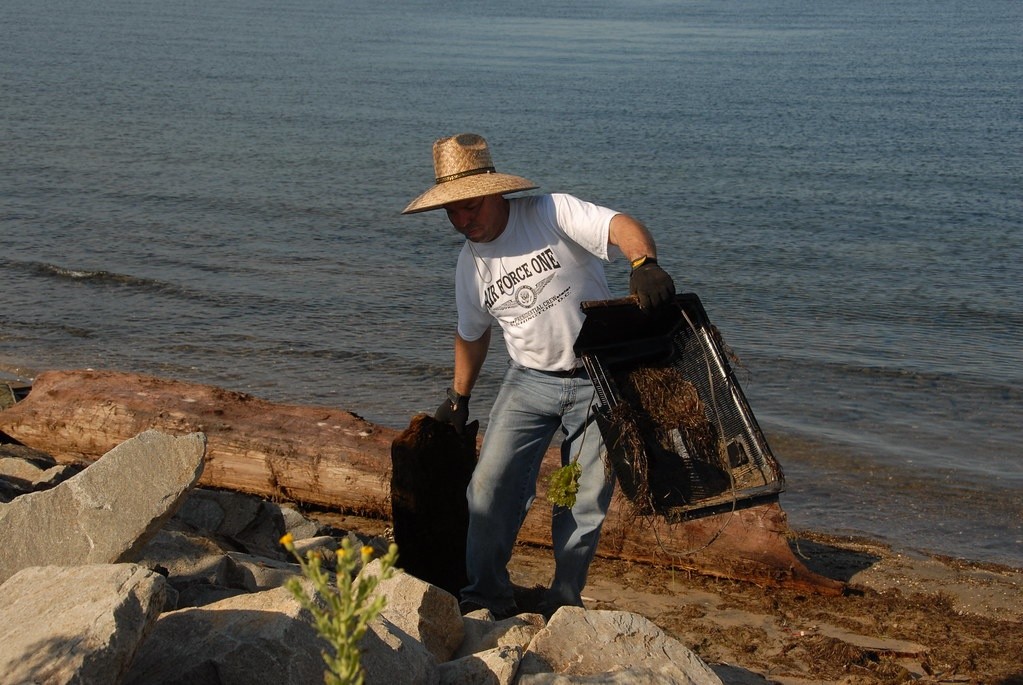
399, 133, 677, 621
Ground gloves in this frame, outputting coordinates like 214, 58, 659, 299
434, 389, 472, 435
630, 254, 677, 312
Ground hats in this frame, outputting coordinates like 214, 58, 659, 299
401, 132, 540, 214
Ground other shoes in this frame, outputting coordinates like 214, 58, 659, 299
460, 600, 519, 620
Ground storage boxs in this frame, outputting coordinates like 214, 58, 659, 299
388, 418, 479, 605
575, 292, 789, 526
